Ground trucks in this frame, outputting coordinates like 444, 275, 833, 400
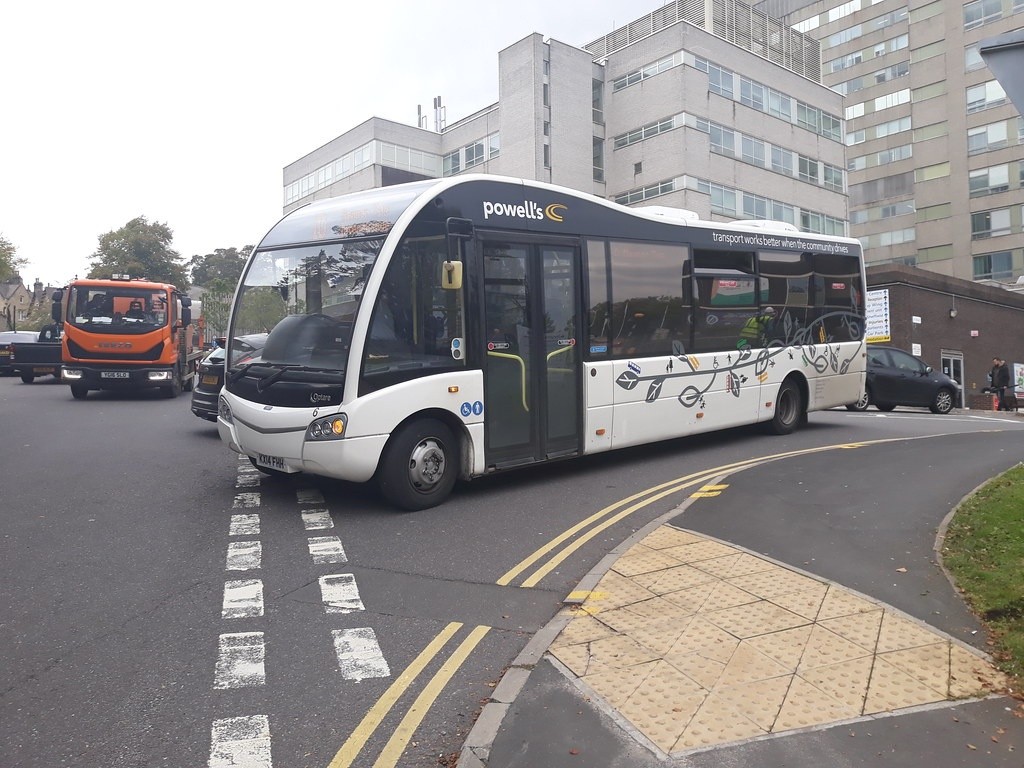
50, 273, 213, 398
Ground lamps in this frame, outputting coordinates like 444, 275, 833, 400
949, 307, 958, 317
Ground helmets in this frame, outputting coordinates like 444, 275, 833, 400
764, 307, 776, 317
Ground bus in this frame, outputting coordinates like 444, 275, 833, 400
213, 173, 870, 513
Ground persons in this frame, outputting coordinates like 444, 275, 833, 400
329, 276, 397, 348
125, 303, 146, 319
989, 356, 1011, 411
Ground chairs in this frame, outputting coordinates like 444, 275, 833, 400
388, 283, 412, 339
126, 301, 145, 317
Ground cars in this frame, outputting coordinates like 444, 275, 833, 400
0, 330, 66, 376
846, 345, 961, 414
190, 332, 272, 423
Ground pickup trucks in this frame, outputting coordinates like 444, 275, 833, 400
9, 338, 63, 383
